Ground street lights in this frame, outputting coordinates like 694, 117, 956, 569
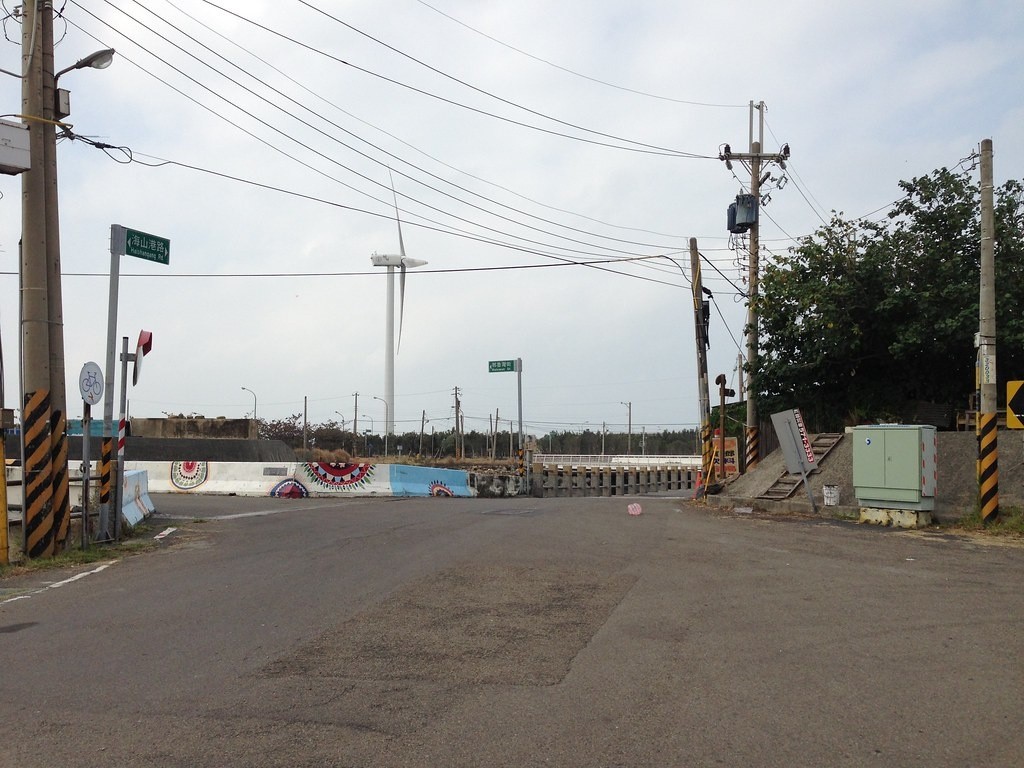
41, 45, 118, 555
241, 386, 258, 419
362, 413, 374, 436
369, 253, 427, 432
621, 401, 632, 452
451, 405, 466, 460
372, 395, 389, 458
335, 411, 345, 448
